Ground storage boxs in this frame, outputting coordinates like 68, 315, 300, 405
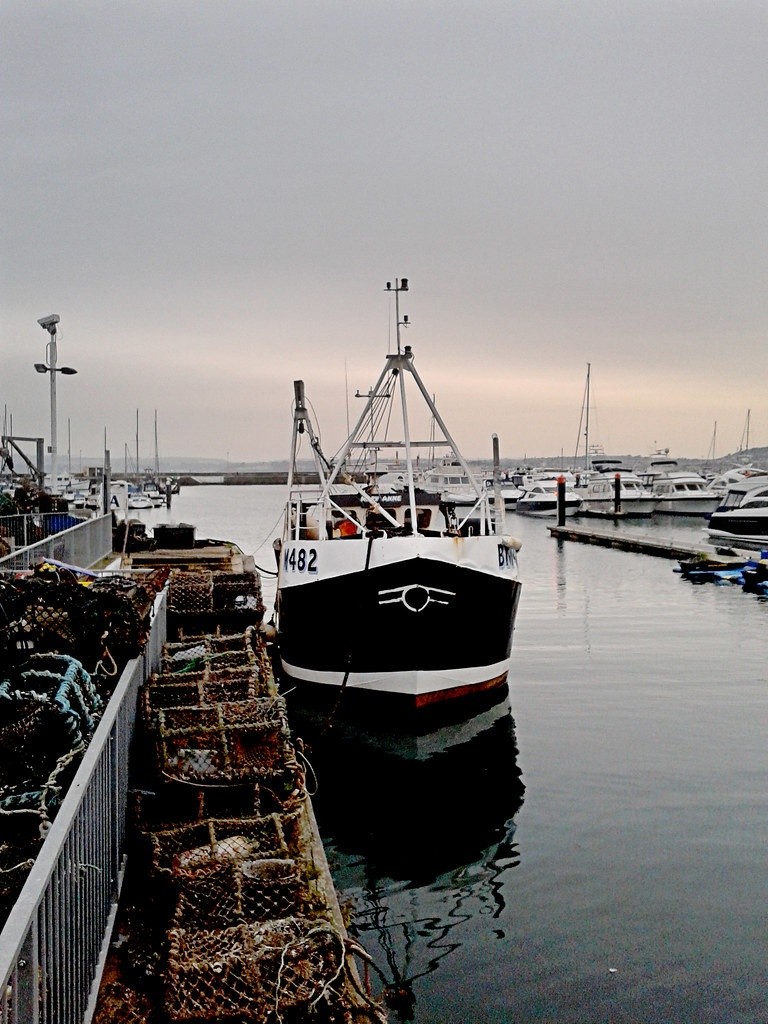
2, 560, 353, 1024
154, 526, 195, 548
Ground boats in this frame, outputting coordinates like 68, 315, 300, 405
277, 274, 524, 709
1, 315, 202, 521
370, 364, 768, 555
